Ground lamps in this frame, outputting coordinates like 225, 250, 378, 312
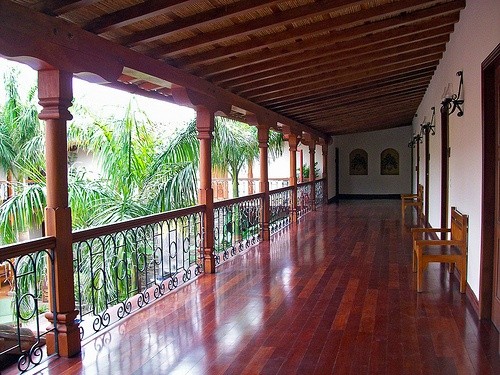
414, 133, 423, 144
440, 70, 464, 117
419, 106, 435, 135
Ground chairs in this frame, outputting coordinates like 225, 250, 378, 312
410, 206, 468, 295
400, 184, 424, 226
